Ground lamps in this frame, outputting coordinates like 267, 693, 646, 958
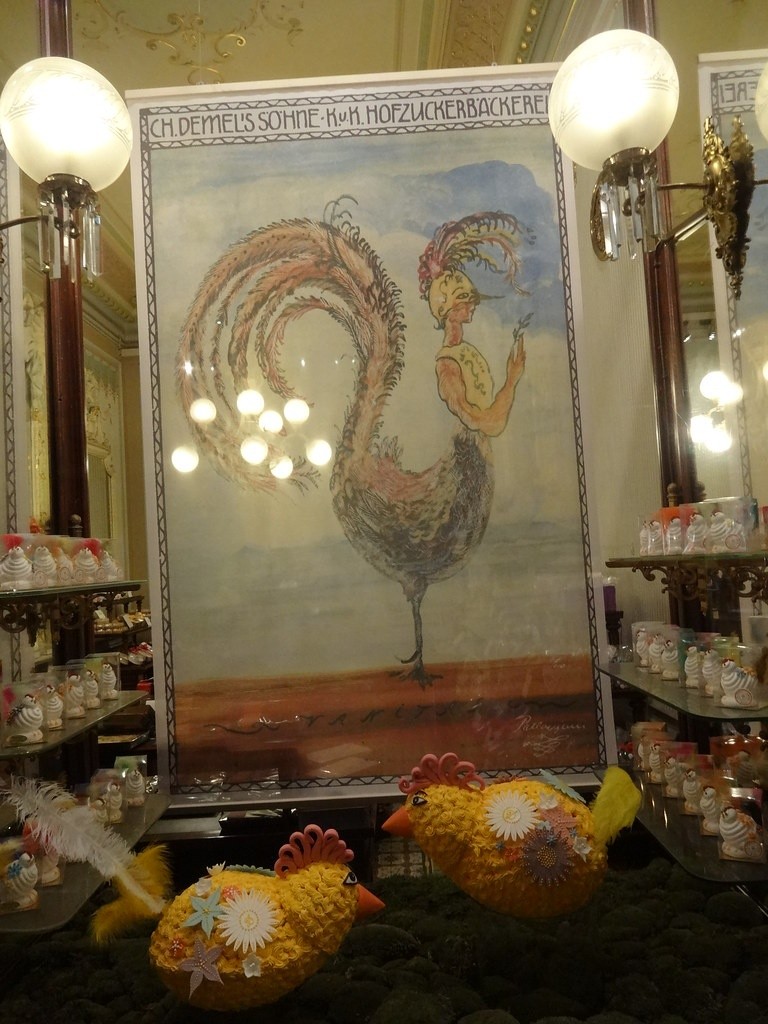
549, 29, 768, 261
0, 55, 133, 280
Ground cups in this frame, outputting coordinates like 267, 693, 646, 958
0, 756, 148, 912
630, 621, 768, 710
631, 721, 768, 863
1, 652, 120, 744
638, 496, 761, 557
0, 533, 124, 591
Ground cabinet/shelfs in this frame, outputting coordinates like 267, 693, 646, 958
593, 550, 768, 883
97, 588, 156, 773
0, 577, 172, 934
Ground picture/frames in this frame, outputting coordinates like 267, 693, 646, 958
124, 62, 618, 810
